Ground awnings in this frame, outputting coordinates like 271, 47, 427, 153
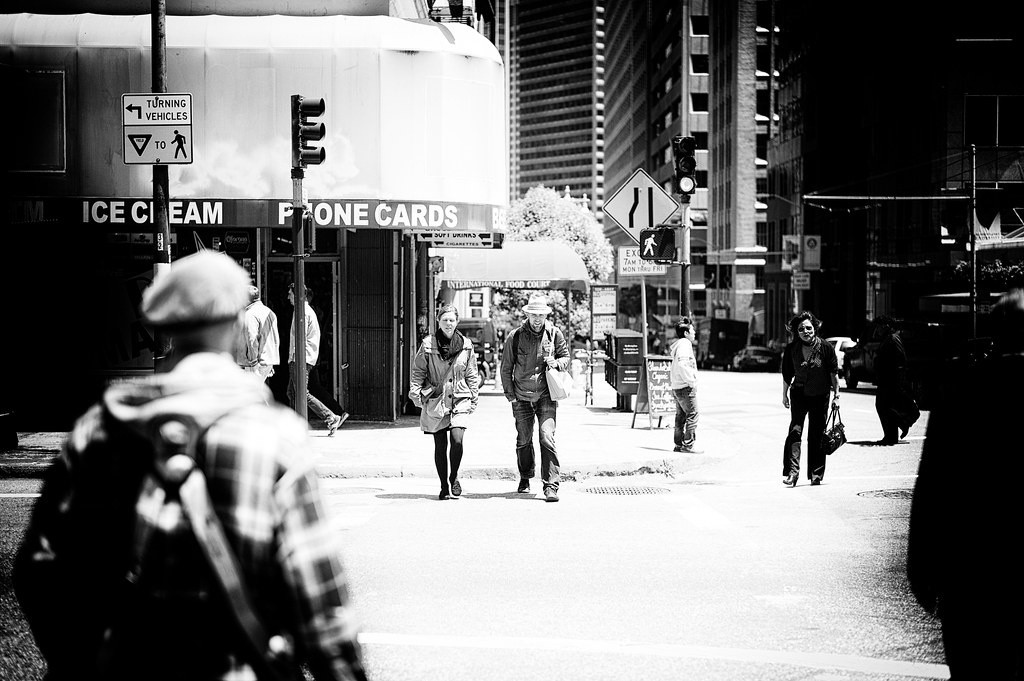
429, 237, 584, 378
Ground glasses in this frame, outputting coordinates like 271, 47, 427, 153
797, 325, 813, 333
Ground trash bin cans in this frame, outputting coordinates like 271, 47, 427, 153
604, 329, 643, 412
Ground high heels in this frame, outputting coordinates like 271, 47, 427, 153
811, 478, 820, 485
783, 475, 798, 487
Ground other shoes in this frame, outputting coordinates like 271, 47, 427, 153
439, 489, 450, 500
901, 412, 920, 439
518, 479, 530, 493
674, 445, 697, 453
449, 477, 462, 496
544, 488, 559, 502
877, 440, 893, 444
328, 415, 341, 436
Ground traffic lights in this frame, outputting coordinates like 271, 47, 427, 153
670, 134, 697, 193
291, 95, 326, 168
638, 227, 675, 260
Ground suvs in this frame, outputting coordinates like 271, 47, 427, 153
841, 319, 905, 390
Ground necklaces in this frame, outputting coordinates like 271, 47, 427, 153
799, 339, 815, 347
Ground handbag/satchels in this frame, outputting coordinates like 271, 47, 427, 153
545, 326, 573, 401
420, 378, 437, 403
821, 409, 847, 455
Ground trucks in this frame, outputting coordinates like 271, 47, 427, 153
692, 316, 750, 372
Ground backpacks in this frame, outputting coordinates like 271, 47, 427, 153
93, 405, 290, 681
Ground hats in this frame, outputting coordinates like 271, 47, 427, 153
141, 250, 251, 325
522, 293, 552, 314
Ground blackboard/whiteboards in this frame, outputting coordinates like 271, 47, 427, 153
635, 356, 677, 415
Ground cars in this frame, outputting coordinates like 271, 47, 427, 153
824, 336, 857, 371
733, 346, 774, 372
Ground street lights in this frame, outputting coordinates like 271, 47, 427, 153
756, 192, 806, 314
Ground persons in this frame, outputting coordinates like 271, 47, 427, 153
781, 311, 839, 488
285, 282, 349, 436
12, 249, 367, 681
668, 316, 705, 454
410, 304, 479, 499
873, 322, 919, 446
501, 295, 571, 503
906, 311, 1024, 681
236, 285, 280, 386
491, 304, 510, 341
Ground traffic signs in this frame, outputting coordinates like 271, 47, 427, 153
120, 92, 194, 167
